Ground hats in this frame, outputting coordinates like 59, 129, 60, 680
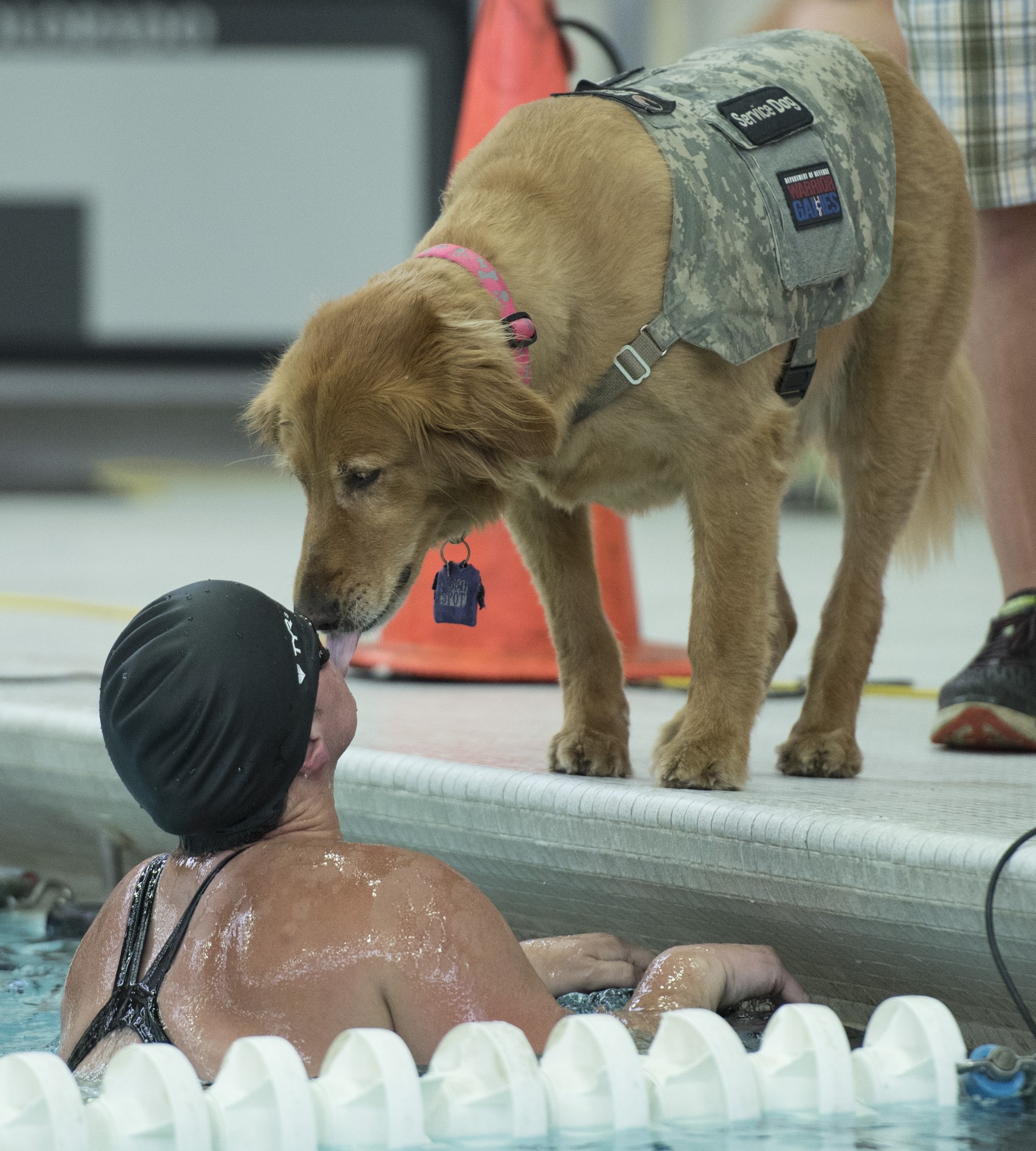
100, 579, 317, 848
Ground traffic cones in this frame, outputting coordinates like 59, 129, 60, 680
315, 3, 717, 682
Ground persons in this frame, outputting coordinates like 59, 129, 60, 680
55, 578, 810, 1087
900, 0, 1036, 754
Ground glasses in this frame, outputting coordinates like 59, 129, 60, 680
298, 613, 331, 671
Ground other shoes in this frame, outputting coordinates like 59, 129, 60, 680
927, 589, 1036, 750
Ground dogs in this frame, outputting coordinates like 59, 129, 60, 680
240, 26, 990, 794
